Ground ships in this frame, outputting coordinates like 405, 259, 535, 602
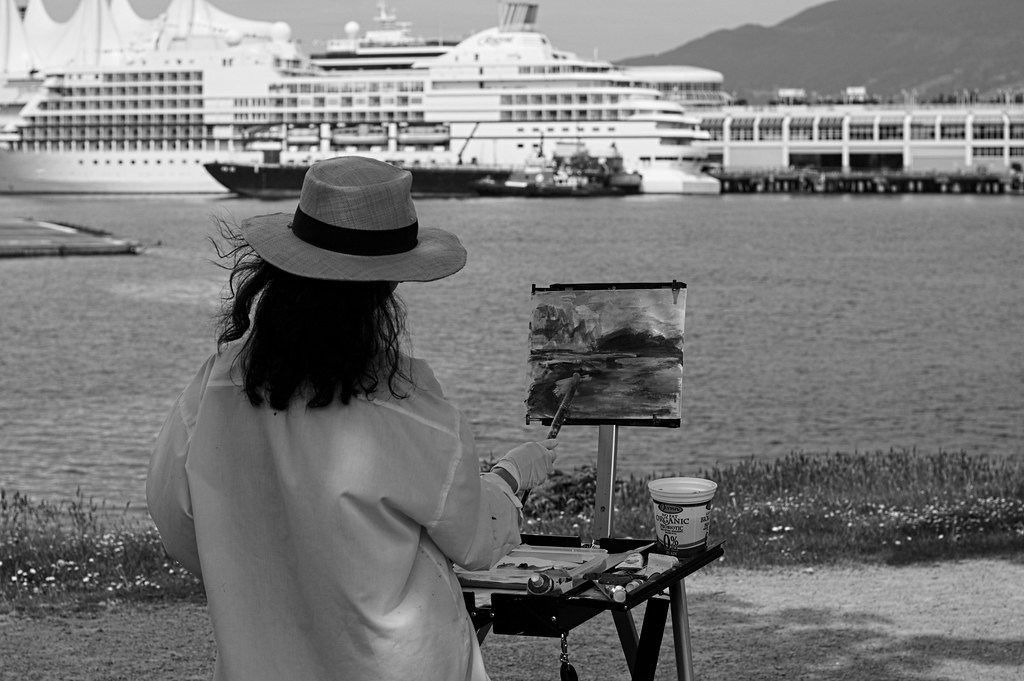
0, 0, 721, 198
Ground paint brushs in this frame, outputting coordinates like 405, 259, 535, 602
518, 370, 580, 512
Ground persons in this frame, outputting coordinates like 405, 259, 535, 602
146, 156, 559, 681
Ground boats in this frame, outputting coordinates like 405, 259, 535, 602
203, 117, 509, 199
472, 136, 643, 198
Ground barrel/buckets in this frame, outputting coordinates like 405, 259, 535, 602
647, 477, 718, 550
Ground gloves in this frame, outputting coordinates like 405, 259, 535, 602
489, 439, 559, 495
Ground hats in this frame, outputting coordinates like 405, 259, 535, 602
241, 156, 467, 282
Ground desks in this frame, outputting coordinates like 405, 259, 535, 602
454, 533, 726, 680
